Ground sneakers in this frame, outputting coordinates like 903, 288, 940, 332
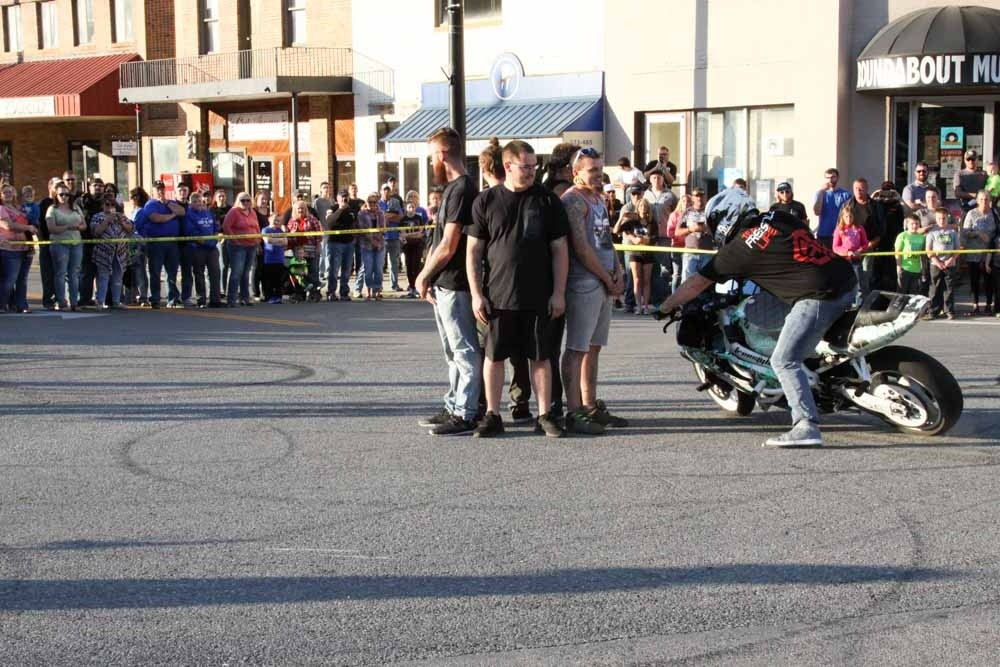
417, 397, 628, 440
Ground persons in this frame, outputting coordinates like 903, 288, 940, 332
598, 143, 717, 315
387, 176, 443, 297
895, 148, 1000, 321
845, 167, 912, 293
769, 180, 809, 227
36, 170, 261, 308
735, 179, 747, 191
410, 126, 628, 439
253, 180, 403, 304
646, 184, 860, 446
0, 175, 40, 314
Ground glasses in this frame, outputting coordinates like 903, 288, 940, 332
63, 178, 75, 181
779, 190, 790, 194
239, 199, 251, 202
367, 201, 377, 204
56, 192, 70, 197
573, 147, 596, 166
606, 190, 614, 193
93, 183, 103, 187
916, 170, 928, 173
632, 192, 642, 196
966, 158, 975, 161
509, 162, 540, 171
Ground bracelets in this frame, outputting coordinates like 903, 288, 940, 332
659, 305, 671, 313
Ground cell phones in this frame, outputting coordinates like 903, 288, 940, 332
879, 191, 892, 197
659, 156, 664, 163
344, 199, 347, 204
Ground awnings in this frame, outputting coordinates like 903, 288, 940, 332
0, 54, 145, 121
855, 5, 1000, 98
378, 100, 602, 163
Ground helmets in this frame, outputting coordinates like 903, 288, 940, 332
704, 186, 758, 250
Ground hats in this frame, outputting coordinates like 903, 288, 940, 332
630, 186, 644, 193
338, 190, 349, 196
965, 150, 977, 160
603, 184, 615, 192
294, 189, 304, 197
152, 180, 164, 187
776, 182, 790, 191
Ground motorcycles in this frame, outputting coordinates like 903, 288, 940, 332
651, 268, 963, 436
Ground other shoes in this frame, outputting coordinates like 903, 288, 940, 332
765, 420, 823, 446
0, 284, 420, 314
921, 303, 1000, 320
